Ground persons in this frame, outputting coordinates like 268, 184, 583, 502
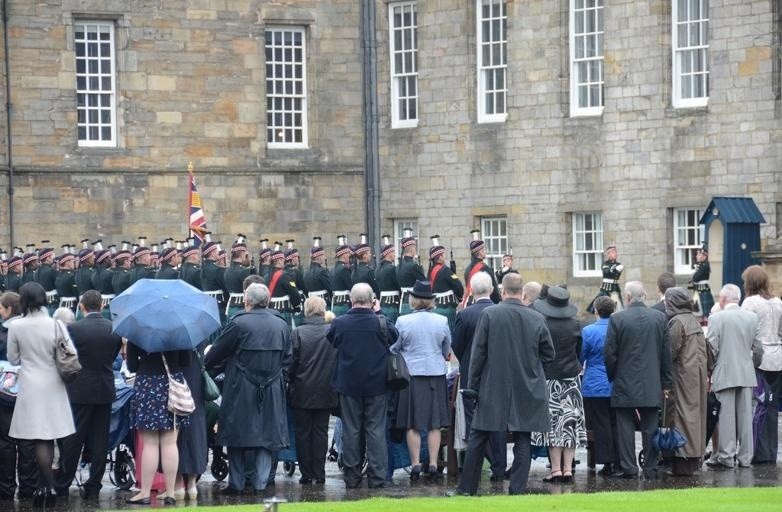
1, 228, 782, 510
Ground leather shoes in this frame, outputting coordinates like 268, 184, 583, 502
156, 487, 198, 504
124, 497, 151, 505
444, 488, 473, 497
222, 486, 266, 496
706, 461, 734, 470
299, 476, 325, 483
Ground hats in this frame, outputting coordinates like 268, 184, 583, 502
407, 280, 437, 299
470, 240, 485, 254
607, 246, 616, 253
696, 248, 708, 256
310, 236, 445, 259
0, 242, 300, 269
502, 254, 512, 261
532, 286, 578, 319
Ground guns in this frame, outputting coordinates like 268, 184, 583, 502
450, 239, 455, 271
349, 255, 357, 279
298, 257, 303, 275
251, 252, 257, 274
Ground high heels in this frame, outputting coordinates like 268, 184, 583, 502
32, 486, 58, 508
410, 465, 443, 483
598, 462, 616, 476
543, 469, 573, 483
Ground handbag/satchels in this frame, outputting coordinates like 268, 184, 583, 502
386, 352, 411, 393
167, 377, 196, 417
0, 359, 21, 402
201, 370, 220, 402
55, 340, 82, 377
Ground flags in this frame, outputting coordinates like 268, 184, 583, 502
189, 168, 211, 249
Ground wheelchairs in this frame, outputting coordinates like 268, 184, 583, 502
73, 437, 664, 499
